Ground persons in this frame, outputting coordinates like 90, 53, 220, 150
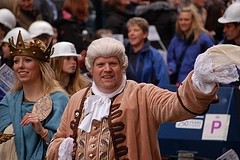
46, 37, 240, 160
0, 30, 69, 160
0, 0, 240, 101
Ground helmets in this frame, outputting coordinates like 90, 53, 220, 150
218, 2, 240, 24
29, 21, 54, 38
50, 41, 82, 58
2, 27, 31, 44
0, 8, 16, 29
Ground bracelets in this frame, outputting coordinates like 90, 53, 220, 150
42, 130, 48, 138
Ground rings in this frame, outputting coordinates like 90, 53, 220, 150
30, 115, 31, 118
